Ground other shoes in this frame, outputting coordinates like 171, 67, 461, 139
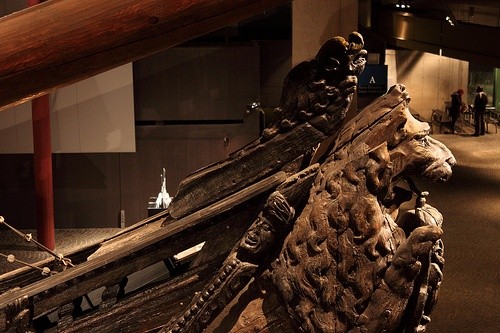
471, 133, 479, 136
453, 130, 457, 133
480, 132, 484, 135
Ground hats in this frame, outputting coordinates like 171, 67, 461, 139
459, 88, 464, 94
476, 85, 483, 93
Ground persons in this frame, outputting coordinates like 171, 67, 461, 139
160, 190, 298, 332
472, 85, 488, 136
450, 89, 465, 131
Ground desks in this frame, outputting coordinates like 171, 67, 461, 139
444, 101, 478, 135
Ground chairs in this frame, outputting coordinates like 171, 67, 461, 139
430, 108, 452, 134
